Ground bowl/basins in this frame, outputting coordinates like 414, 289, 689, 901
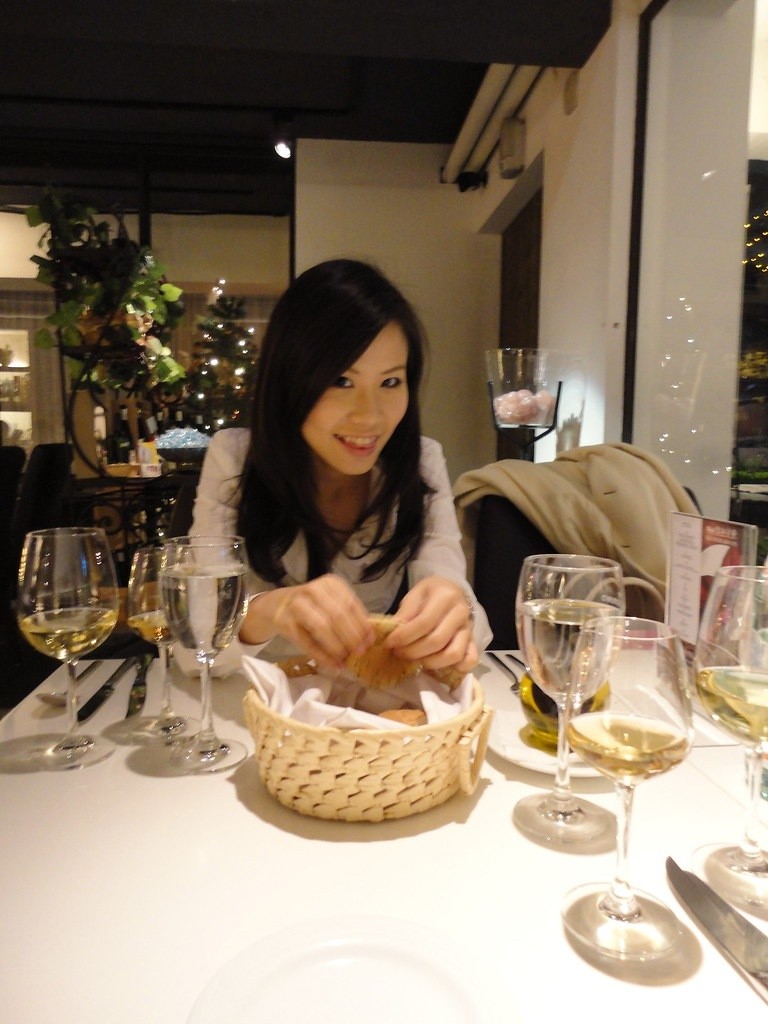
106, 464, 132, 476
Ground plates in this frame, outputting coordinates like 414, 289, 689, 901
487, 697, 613, 778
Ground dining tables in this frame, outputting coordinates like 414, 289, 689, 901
0, 652, 768, 1024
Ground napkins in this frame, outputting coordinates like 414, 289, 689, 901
239, 655, 475, 745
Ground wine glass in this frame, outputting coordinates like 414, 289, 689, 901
561, 616, 695, 961
512, 553, 626, 852
16, 527, 120, 769
124, 547, 209, 745
157, 534, 250, 773
686, 565, 768, 909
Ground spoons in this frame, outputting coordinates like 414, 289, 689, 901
486, 652, 521, 694
36, 660, 103, 708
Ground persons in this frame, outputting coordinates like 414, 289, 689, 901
185, 257, 495, 675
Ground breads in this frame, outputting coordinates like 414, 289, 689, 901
302, 618, 465, 726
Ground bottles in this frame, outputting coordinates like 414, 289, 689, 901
11, 376, 22, 404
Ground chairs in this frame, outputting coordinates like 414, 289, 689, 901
0, 446, 28, 544
20, 442, 74, 546
456, 443, 704, 652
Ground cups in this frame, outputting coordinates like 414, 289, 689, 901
140, 464, 161, 477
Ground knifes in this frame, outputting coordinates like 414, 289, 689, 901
125, 653, 155, 719
77, 656, 137, 722
665, 856, 768, 1007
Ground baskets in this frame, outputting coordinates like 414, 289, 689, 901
245, 612, 493, 824
